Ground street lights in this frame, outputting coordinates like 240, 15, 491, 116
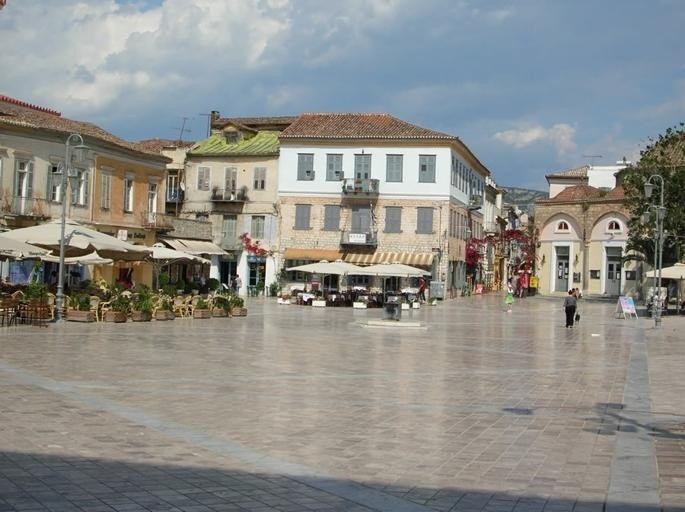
644, 170, 666, 328
55, 130, 92, 322
642, 205, 658, 321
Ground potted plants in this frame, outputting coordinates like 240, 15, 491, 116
231, 296, 248, 316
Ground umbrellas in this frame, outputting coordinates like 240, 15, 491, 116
645, 262, 685, 315
284, 258, 432, 309
1, 216, 214, 291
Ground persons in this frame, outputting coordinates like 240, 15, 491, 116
505, 271, 685, 330
230, 275, 238, 292
234, 274, 244, 293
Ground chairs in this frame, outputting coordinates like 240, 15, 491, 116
299, 288, 417, 309
0, 289, 231, 327
